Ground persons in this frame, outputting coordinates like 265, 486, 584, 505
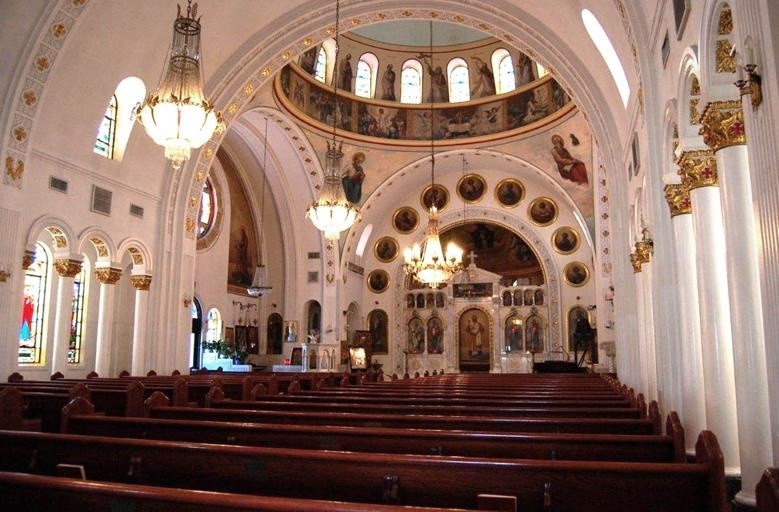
550, 135, 587, 186
428, 190, 444, 210
400, 211, 411, 230
570, 266, 583, 284
430, 321, 442, 352
411, 321, 424, 351
372, 274, 385, 290
537, 202, 552, 218
464, 179, 481, 198
340, 153, 365, 203
502, 184, 517, 202
380, 242, 393, 259
466, 314, 482, 355
574, 310, 590, 334
530, 319, 540, 349
281, 46, 571, 139
558, 233, 572, 251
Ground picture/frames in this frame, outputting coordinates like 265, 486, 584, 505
367, 173, 590, 294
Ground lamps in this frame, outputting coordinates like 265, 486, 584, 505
129, 0, 224, 169
246, 119, 274, 297
306, 0, 362, 245
402, 21, 463, 291
